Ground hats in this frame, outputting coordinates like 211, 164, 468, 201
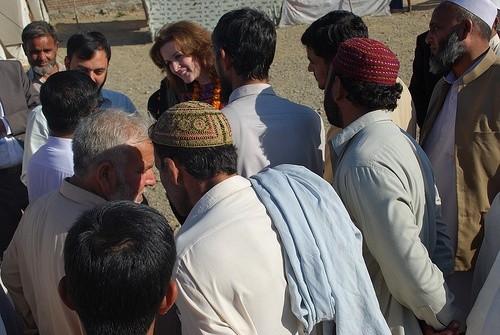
447, 0, 497, 29
151, 101, 234, 147
332, 38, 401, 85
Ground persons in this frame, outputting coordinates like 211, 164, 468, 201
153, 100, 392, 335
407, 28, 449, 127
488, 0, 500, 61
301, 9, 417, 188
19, 20, 68, 99
26, 69, 102, 215
211, 7, 326, 183
323, 38, 467, 335
416, 0, 499, 275
0, 110, 157, 335
146, 19, 230, 120
57, 200, 179, 335
0, 59, 42, 256
21, 30, 141, 188
420, 192, 500, 335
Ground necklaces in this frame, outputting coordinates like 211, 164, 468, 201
191, 70, 221, 110
